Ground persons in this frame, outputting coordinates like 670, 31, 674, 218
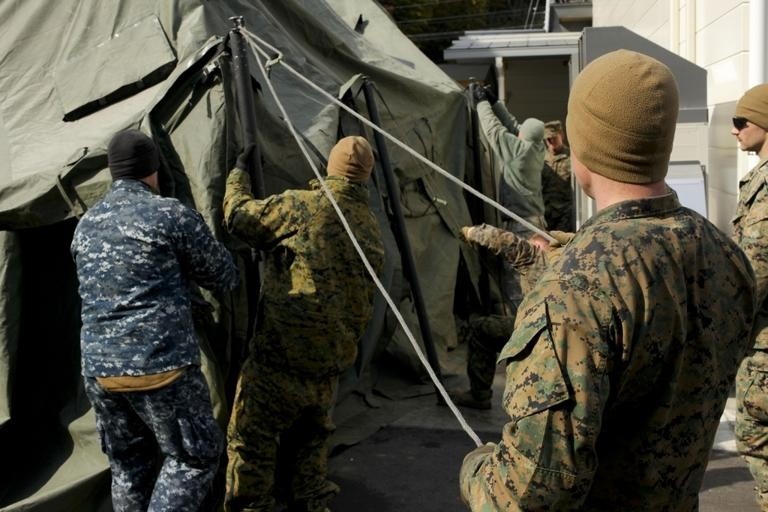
458, 48, 759, 512
467, 77, 549, 312
542, 118, 575, 230
69, 130, 242, 512
221, 135, 386, 512
451, 223, 566, 411
730, 83, 768, 512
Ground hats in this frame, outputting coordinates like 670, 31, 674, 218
519, 118, 545, 144
544, 120, 563, 138
327, 135, 375, 183
109, 129, 159, 181
735, 85, 768, 129
567, 49, 678, 184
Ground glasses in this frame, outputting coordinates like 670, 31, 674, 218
733, 117, 747, 130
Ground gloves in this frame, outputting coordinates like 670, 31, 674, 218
236, 142, 259, 171
473, 82, 498, 105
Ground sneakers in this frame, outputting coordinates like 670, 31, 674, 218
447, 387, 492, 410
